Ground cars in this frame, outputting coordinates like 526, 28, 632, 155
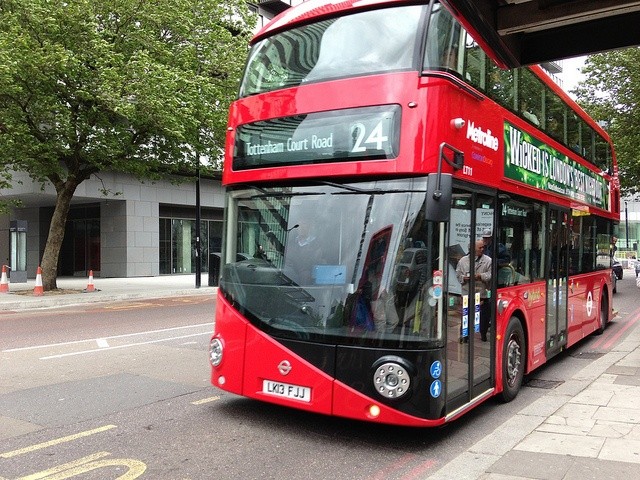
612, 255, 623, 280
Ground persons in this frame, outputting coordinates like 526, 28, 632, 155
440, 46, 471, 80
545, 115, 562, 144
612, 235, 618, 256
456, 241, 493, 344
288, 216, 325, 284
520, 95, 541, 127
568, 130, 581, 154
366, 270, 400, 336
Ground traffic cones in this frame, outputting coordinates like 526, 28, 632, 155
0, 264, 9, 292
87, 270, 95, 292
33, 266, 44, 296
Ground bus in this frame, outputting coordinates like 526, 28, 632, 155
210, 0, 620, 427
